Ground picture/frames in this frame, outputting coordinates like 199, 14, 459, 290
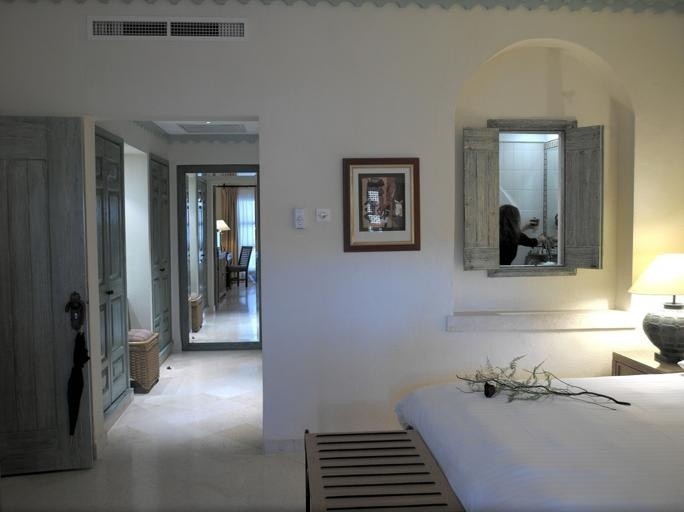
342, 158, 420, 252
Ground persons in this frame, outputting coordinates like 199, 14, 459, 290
500, 204, 549, 265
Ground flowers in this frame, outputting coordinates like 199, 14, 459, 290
455, 352, 631, 407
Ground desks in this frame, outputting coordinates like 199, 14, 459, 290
218, 252, 227, 303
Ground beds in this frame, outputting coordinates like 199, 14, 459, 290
394, 373, 684, 512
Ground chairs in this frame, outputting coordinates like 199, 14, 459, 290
226, 246, 252, 290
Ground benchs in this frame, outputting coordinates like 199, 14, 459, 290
304, 425, 467, 512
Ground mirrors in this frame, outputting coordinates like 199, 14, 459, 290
176, 164, 262, 351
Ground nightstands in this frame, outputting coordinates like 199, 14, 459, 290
612, 348, 684, 376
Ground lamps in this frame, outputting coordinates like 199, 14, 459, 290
626, 253, 684, 364
216, 219, 231, 254
